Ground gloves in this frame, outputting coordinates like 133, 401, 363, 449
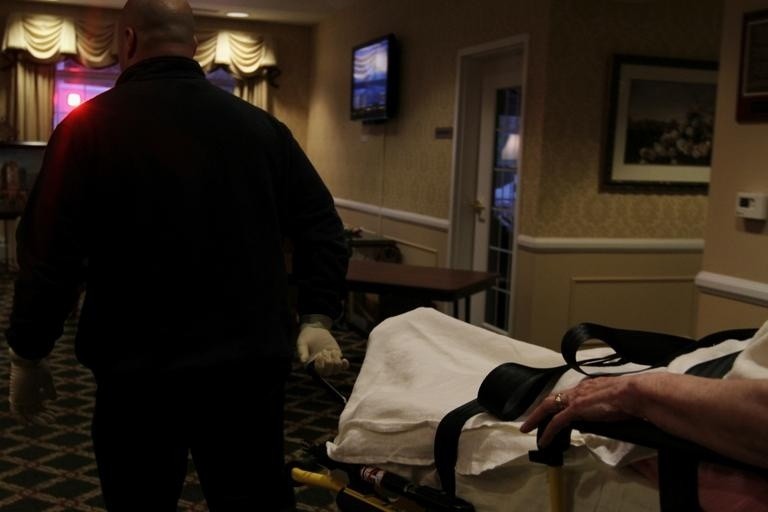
295, 313, 350, 377
7, 347, 60, 431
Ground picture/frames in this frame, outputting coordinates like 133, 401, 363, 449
605, 54, 719, 188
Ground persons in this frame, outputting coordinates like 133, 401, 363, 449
520, 319, 768, 469
8, 0, 348, 512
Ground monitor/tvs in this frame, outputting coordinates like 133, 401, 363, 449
350, 32, 401, 125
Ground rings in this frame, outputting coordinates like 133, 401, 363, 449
554, 392, 564, 408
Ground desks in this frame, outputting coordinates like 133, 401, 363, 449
284, 251, 496, 324
